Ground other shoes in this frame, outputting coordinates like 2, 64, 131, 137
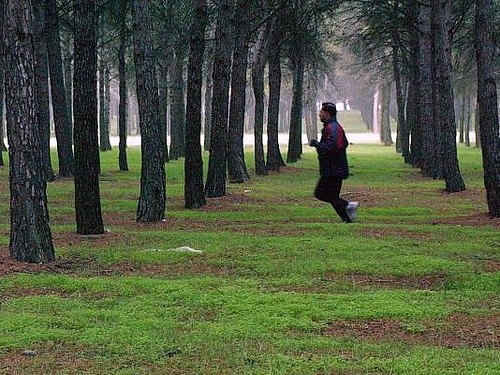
346, 199, 360, 222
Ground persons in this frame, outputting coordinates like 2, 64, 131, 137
308, 101, 360, 222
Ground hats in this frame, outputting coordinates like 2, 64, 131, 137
322, 102, 336, 116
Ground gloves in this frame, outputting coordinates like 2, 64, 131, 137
309, 139, 319, 147
